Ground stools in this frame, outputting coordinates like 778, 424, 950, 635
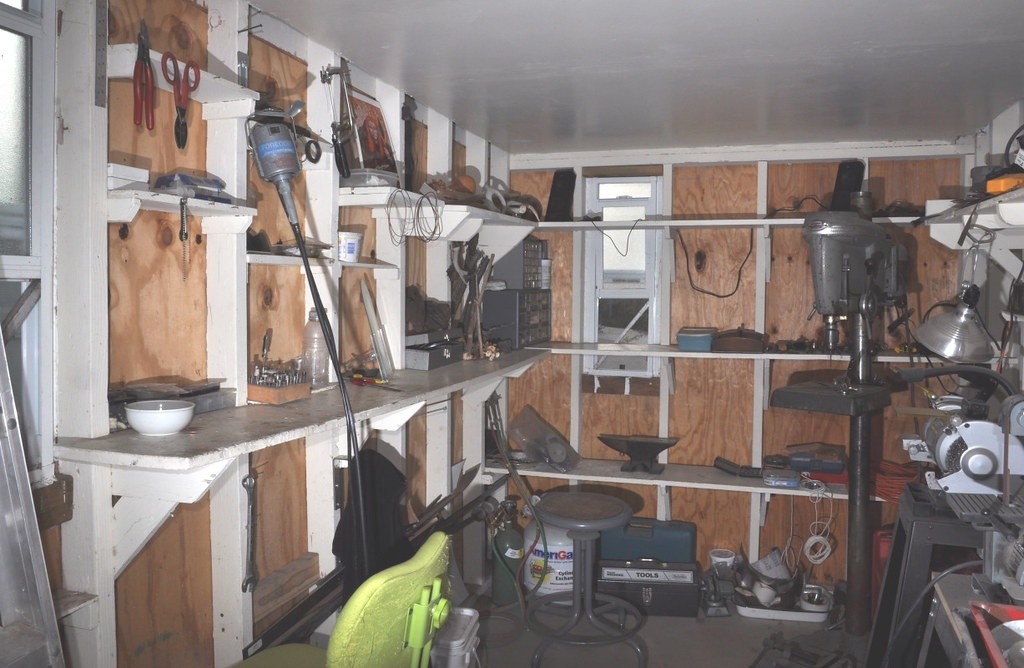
525, 492, 646, 668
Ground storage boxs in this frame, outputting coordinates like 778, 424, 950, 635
676, 327, 716, 352
340, 168, 398, 188
593, 560, 707, 618
249, 382, 311, 404
431, 608, 481, 668
406, 327, 464, 370
108, 176, 150, 192
108, 161, 149, 183
186, 387, 237, 413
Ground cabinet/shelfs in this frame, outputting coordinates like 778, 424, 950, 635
486, 188, 1024, 503
105, 42, 538, 271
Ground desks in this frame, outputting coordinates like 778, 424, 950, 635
53, 348, 547, 668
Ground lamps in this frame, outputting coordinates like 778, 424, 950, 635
917, 283, 994, 363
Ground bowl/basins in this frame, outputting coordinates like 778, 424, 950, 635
337, 232, 363, 262
800, 593, 830, 612
123, 400, 196, 437
338, 168, 399, 187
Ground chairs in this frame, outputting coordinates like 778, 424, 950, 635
230, 532, 449, 668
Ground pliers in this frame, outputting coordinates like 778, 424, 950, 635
350, 378, 374, 386
134, 20, 154, 130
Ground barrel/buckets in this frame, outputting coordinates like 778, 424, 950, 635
752, 547, 791, 582
708, 548, 736, 575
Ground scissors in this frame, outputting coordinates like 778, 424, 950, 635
162, 50, 200, 149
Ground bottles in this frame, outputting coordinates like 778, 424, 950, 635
302, 310, 329, 390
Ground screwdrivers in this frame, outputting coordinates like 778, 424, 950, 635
347, 374, 362, 378
